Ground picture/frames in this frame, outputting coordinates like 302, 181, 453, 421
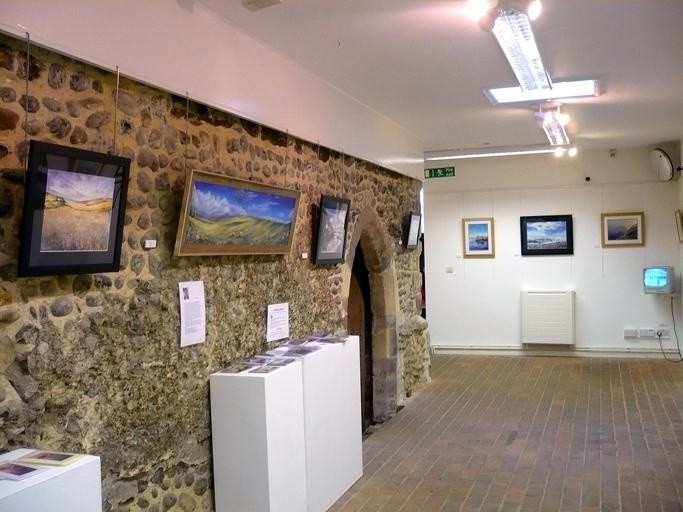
172, 167, 302, 258
461, 211, 646, 259
310, 192, 352, 268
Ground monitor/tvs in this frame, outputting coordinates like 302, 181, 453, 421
641, 265, 675, 294
315, 194, 351, 265
405, 211, 422, 250
